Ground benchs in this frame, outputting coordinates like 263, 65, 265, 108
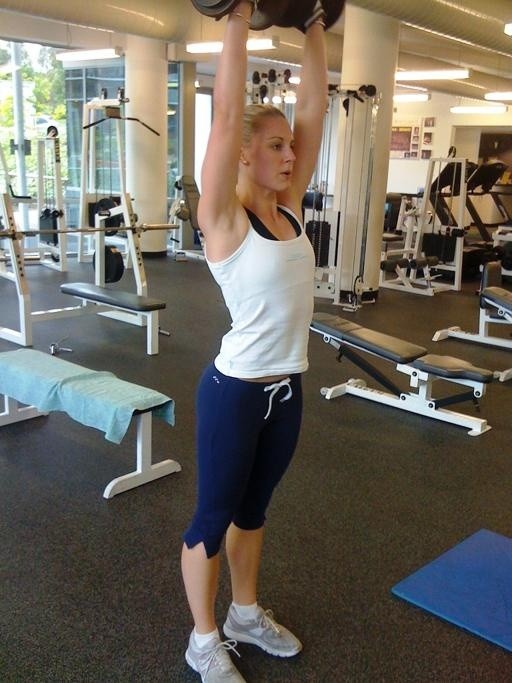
0, 349, 183, 500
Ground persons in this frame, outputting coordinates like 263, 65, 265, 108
178, 3, 328, 683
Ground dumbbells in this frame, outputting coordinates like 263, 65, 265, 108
192, 1, 343, 33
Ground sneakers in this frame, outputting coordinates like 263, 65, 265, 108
223, 607, 302, 658
185, 631, 244, 678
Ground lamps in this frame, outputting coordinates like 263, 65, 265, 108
55, 21, 122, 62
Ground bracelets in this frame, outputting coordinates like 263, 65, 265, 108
311, 18, 327, 28
229, 7, 255, 24
